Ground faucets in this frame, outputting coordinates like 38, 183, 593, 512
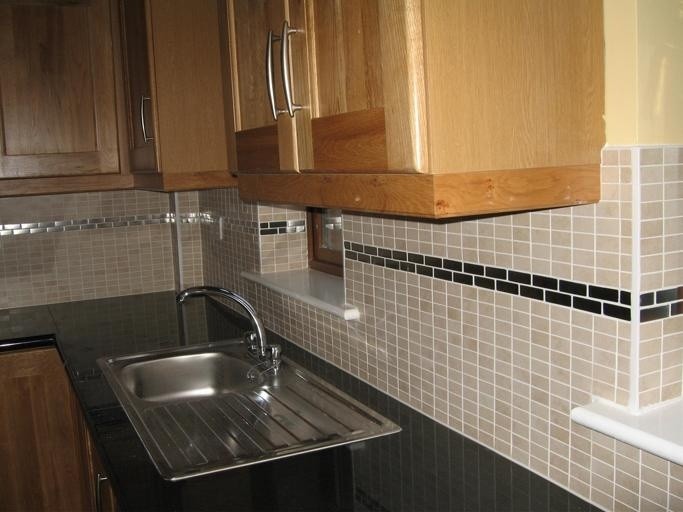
175, 286, 265, 362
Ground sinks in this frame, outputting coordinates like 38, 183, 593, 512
115, 348, 266, 407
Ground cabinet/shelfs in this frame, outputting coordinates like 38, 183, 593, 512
219, 2, 606, 176
119, 0, 230, 177
0, 349, 82, 512
0, 0, 123, 181
72, 399, 119, 512
